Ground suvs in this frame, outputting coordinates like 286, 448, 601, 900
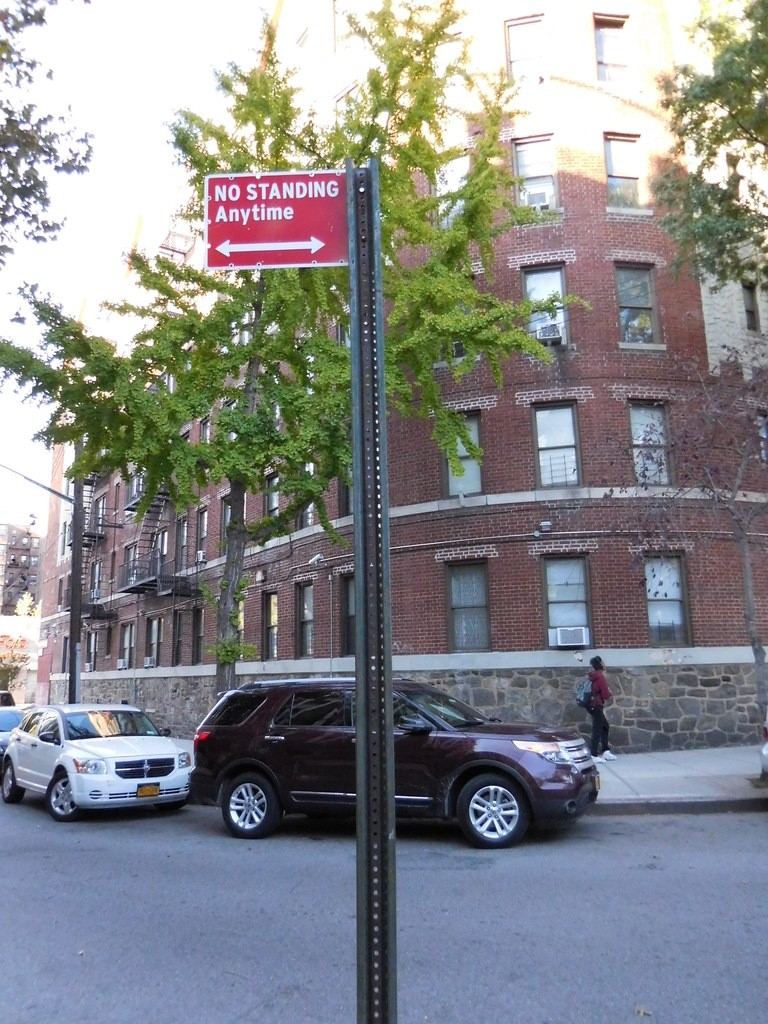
185, 678, 600, 850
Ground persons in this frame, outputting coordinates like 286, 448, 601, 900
585, 655, 617, 763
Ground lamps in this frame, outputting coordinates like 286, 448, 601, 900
539, 521, 552, 532
255, 570, 266, 581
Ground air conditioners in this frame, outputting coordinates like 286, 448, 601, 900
91, 589, 100, 598
536, 322, 562, 341
117, 659, 127, 669
84, 663, 93, 672
57, 605, 64, 613
557, 627, 586, 645
144, 657, 154, 667
195, 551, 206, 561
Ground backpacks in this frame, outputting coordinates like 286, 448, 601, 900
575, 679, 593, 706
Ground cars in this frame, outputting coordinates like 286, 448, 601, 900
0, 707, 26, 764
2, 703, 192, 823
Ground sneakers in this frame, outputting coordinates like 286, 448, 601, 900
592, 756, 606, 764
603, 751, 618, 760
